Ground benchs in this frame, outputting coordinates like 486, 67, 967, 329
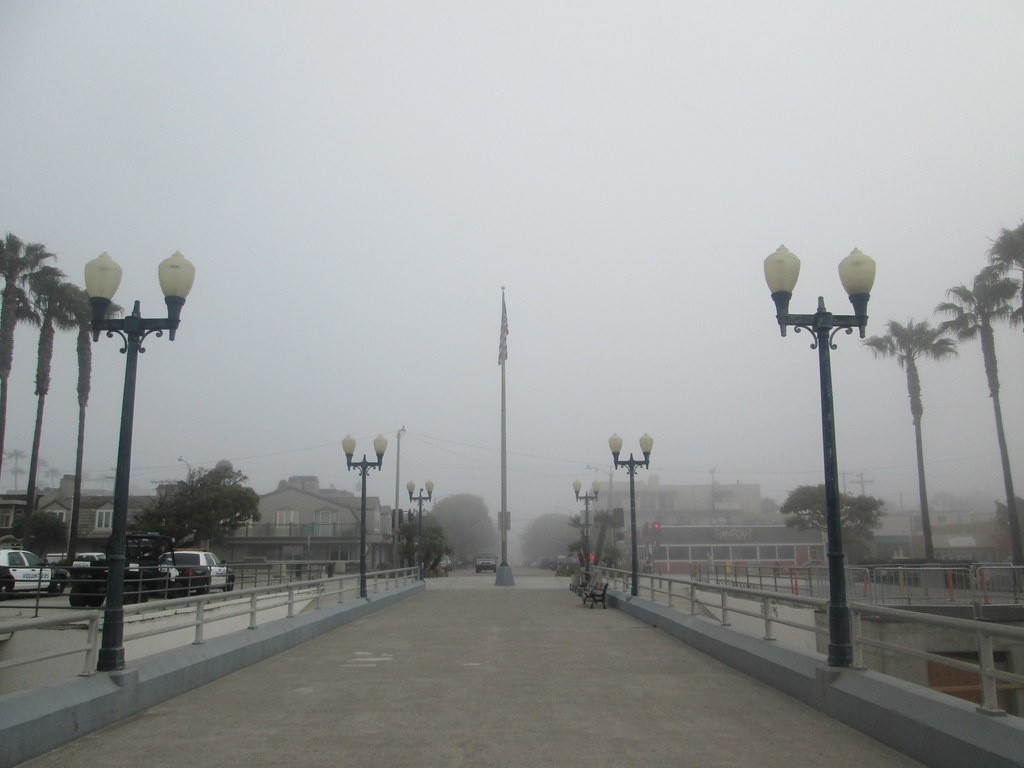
583, 582, 608, 609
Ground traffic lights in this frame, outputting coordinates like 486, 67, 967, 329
653, 522, 659, 531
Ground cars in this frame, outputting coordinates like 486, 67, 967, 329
0, 549, 71, 595
159, 551, 236, 593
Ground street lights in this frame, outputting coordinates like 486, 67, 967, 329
761, 244, 879, 672
341, 434, 389, 599
77, 246, 197, 668
573, 479, 601, 581
406, 480, 433, 578
609, 431, 655, 593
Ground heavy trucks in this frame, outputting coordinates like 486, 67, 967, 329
68, 533, 185, 608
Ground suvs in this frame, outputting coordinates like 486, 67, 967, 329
475, 552, 498, 573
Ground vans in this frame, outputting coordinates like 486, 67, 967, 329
46, 553, 107, 570
